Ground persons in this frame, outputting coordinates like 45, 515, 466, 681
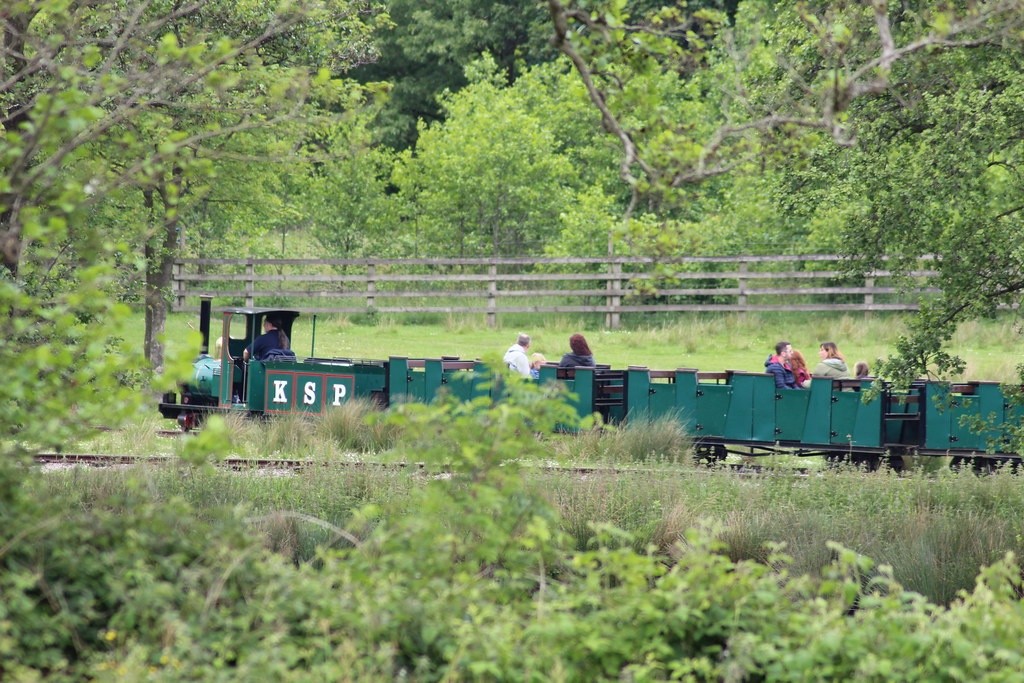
242, 314, 289, 362
503, 333, 530, 377
529, 353, 546, 379
559, 334, 596, 367
765, 342, 800, 390
814, 343, 849, 377
854, 362, 869, 378
789, 350, 810, 387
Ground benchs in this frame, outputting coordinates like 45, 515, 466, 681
557, 367, 727, 409
882, 379, 975, 420
815, 374, 879, 393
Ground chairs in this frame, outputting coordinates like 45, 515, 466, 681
259, 348, 295, 362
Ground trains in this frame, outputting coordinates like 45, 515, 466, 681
155, 297, 1024, 468
